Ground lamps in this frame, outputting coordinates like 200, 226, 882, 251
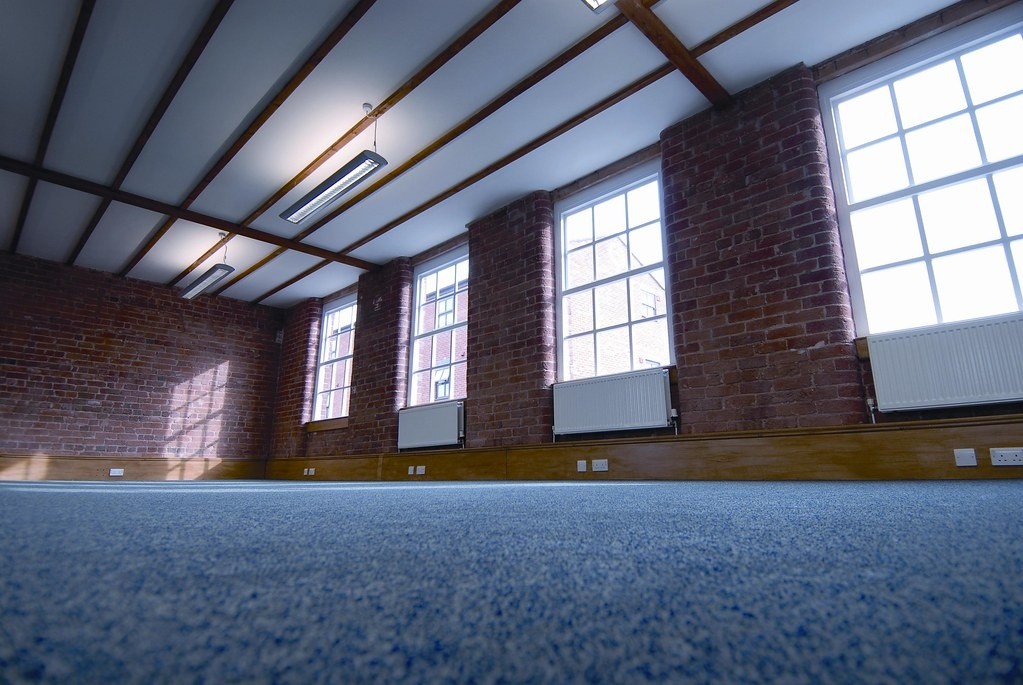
177, 264, 235, 301
279, 150, 388, 224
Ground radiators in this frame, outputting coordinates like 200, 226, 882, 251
552, 368, 678, 442
867, 310, 1023, 413
398, 401, 465, 452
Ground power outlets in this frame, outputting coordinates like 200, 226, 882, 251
417, 466, 425, 474
592, 459, 608, 472
989, 447, 1023, 466
309, 468, 315, 476
